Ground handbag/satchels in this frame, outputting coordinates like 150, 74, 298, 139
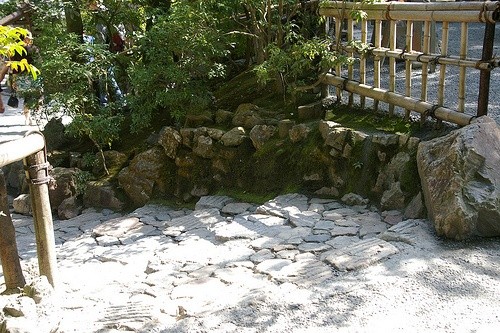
6, 91, 20, 108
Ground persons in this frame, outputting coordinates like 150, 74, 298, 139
0, 0, 134, 127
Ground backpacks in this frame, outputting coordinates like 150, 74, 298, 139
106, 17, 127, 53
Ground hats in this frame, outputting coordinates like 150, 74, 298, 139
20, 29, 34, 40
